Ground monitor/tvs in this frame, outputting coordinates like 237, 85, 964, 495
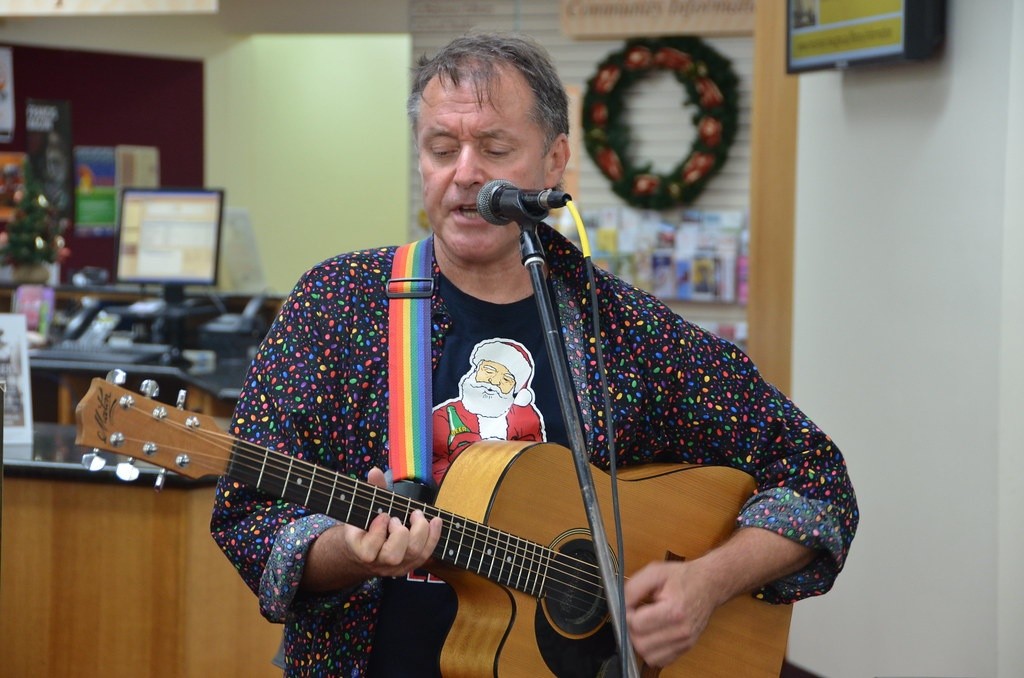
785, 0, 940, 74
113, 187, 224, 287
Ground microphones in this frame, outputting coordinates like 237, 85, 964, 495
478, 181, 573, 226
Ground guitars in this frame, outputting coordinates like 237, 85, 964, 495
74, 368, 793, 678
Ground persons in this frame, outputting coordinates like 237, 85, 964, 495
207, 32, 859, 678
